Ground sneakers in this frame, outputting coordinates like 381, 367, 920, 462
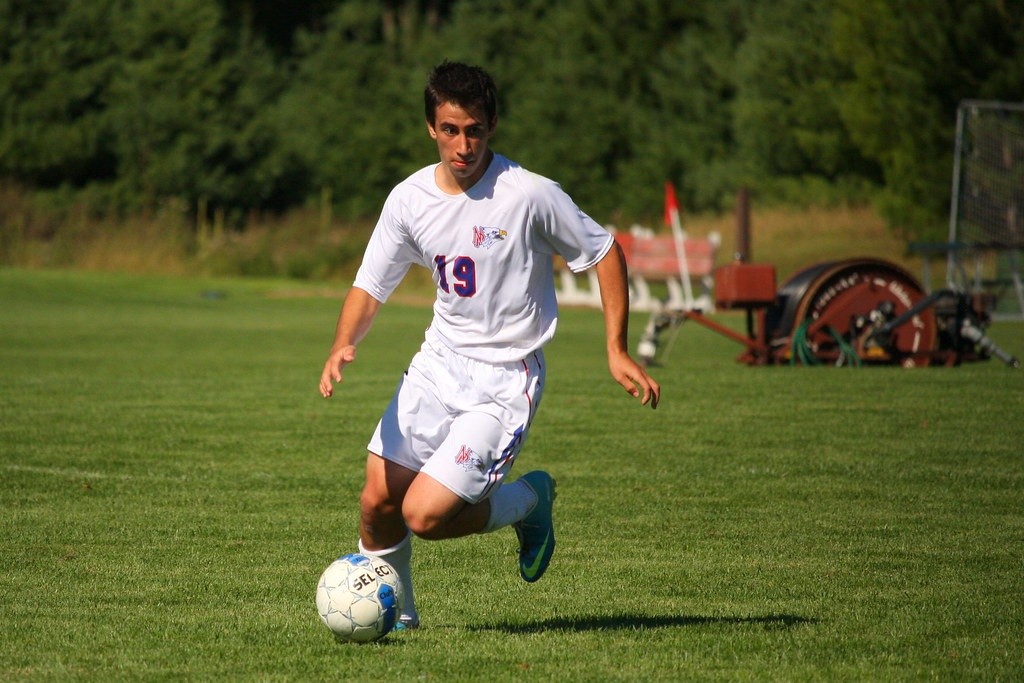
389, 613, 420, 630
511, 471, 558, 582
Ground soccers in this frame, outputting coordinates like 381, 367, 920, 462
316, 553, 403, 641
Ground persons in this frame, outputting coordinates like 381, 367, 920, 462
318, 58, 659, 633
